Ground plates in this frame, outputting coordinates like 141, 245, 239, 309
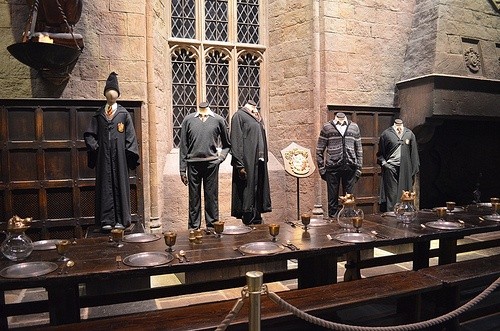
239, 241, 286, 254
122, 233, 162, 243
221, 225, 253, 235
425, 202, 500, 230
0, 261, 60, 278
334, 232, 376, 243
122, 251, 174, 267
32, 239, 63, 251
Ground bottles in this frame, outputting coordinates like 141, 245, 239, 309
311, 205, 324, 222
336, 204, 365, 229
394, 200, 418, 223
0, 231, 34, 261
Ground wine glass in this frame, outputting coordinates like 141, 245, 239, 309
490, 198, 500, 216
213, 221, 224, 238
111, 229, 124, 247
301, 215, 311, 230
55, 240, 73, 261
446, 202, 455, 215
269, 224, 281, 242
352, 217, 363, 233
164, 232, 177, 252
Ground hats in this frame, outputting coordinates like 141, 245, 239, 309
104, 72, 120, 98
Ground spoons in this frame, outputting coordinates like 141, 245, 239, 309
179, 249, 190, 262
64, 260, 74, 273
286, 240, 300, 250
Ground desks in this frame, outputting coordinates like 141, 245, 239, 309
0, 206, 500, 331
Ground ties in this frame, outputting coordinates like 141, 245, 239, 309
108, 106, 112, 118
398, 128, 402, 135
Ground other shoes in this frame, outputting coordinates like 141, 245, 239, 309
103, 225, 112, 230
115, 222, 125, 229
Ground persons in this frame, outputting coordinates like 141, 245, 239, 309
84, 72, 142, 228
230, 100, 272, 226
179, 102, 231, 229
316, 112, 363, 217
376, 118, 420, 213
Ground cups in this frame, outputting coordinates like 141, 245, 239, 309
436, 209, 446, 220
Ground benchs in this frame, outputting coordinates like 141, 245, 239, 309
0, 255, 499, 331
